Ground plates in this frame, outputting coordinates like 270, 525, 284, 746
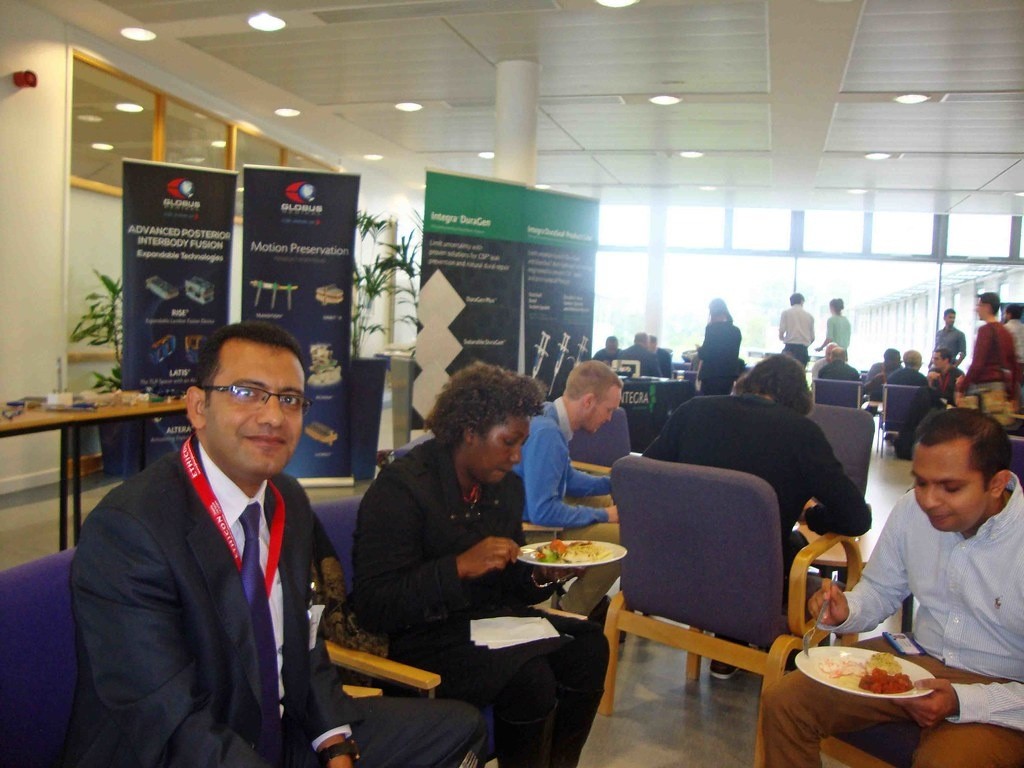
517, 540, 628, 567
794, 645, 936, 699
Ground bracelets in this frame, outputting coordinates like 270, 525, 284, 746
319, 740, 360, 766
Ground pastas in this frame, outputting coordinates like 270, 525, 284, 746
564, 545, 608, 562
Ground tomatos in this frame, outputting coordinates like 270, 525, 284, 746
551, 539, 565, 552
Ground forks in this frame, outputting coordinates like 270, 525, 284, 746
802, 575, 837, 656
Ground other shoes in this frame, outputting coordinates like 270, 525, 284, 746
707, 658, 738, 679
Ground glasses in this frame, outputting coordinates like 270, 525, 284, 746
199, 383, 313, 418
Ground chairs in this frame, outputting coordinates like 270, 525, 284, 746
0, 352, 1023, 767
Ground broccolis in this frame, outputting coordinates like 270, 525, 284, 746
537, 548, 561, 563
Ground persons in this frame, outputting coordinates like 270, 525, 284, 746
511, 361, 627, 644
811, 342, 840, 390
1002, 304, 1024, 399
641, 352, 872, 679
593, 336, 621, 365
649, 335, 672, 380
696, 298, 742, 395
815, 298, 852, 363
762, 408, 1024, 768
61, 322, 488, 768
779, 294, 815, 369
928, 308, 966, 369
884, 350, 928, 430
617, 333, 664, 376
818, 346, 859, 381
864, 348, 903, 416
955, 291, 1020, 401
353, 361, 611, 768
926, 347, 965, 408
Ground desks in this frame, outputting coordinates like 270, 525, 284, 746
0, 391, 187, 570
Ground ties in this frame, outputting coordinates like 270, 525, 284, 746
236, 500, 283, 768
